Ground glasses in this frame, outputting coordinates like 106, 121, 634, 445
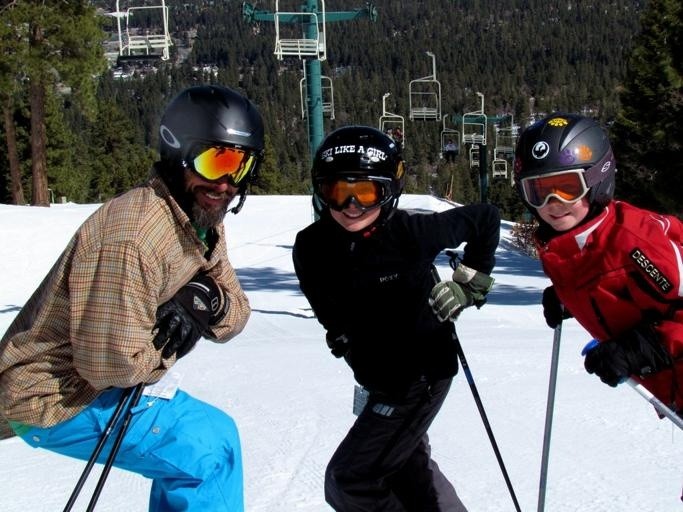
321, 178, 394, 212
520, 168, 591, 210
185, 144, 260, 189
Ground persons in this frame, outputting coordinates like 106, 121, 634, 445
383, 128, 392, 141
292, 122, 502, 511
517, 110, 683, 432
0, 88, 268, 512
444, 135, 459, 168
392, 124, 403, 157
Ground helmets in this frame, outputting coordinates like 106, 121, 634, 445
513, 112, 616, 210
310, 125, 407, 198
155, 84, 268, 162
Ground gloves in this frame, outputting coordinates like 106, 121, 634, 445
541, 286, 573, 330
584, 322, 671, 388
428, 263, 497, 324
151, 273, 222, 362
